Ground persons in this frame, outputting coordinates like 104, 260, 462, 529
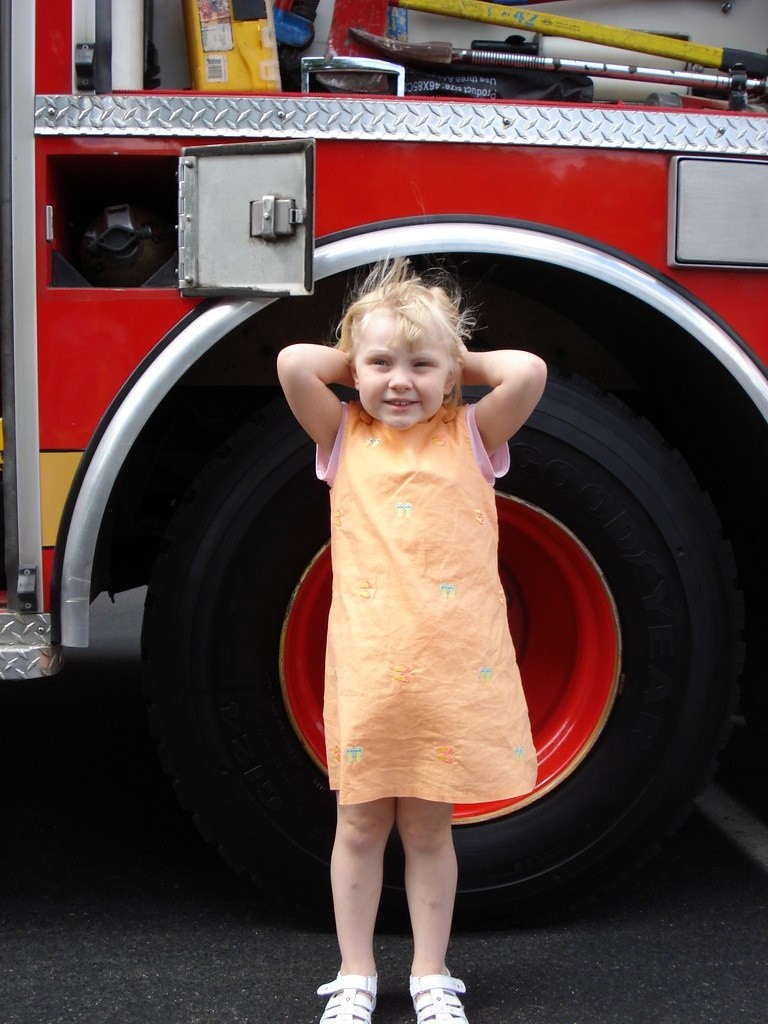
273, 260, 547, 1024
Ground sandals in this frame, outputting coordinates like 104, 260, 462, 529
410, 967, 469, 1024
317, 970, 378, 1024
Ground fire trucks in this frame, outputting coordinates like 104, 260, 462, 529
0, 2, 768, 931
